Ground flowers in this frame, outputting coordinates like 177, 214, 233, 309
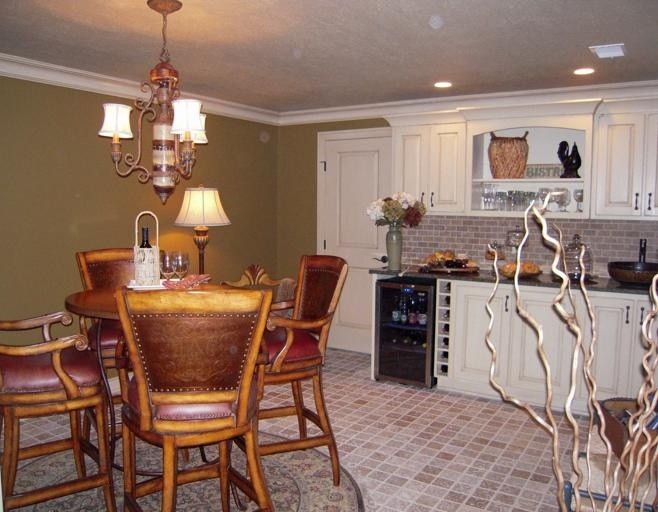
367, 190, 431, 227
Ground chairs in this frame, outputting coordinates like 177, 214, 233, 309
2, 246, 347, 510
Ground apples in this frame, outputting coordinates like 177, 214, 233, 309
502, 261, 539, 275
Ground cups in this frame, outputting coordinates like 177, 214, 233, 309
488, 137, 529, 179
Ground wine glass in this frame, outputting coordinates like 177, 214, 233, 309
170, 251, 189, 281
479, 183, 584, 214
160, 253, 177, 281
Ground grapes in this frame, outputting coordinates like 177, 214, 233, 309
445, 258, 468, 268
419, 260, 445, 272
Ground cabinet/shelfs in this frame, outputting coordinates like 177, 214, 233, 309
457, 92, 606, 220
560, 420, 656, 512
384, 107, 467, 214
574, 287, 658, 427
435, 278, 574, 413
599, 96, 658, 220
372, 275, 433, 392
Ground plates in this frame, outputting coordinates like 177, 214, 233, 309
498, 268, 543, 280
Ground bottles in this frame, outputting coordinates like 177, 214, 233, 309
138, 227, 154, 249
440, 283, 450, 373
392, 291, 428, 326
392, 333, 427, 349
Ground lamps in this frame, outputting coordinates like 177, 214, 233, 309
97, 0, 208, 205
172, 181, 229, 279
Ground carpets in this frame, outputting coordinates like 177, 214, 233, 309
0, 422, 368, 511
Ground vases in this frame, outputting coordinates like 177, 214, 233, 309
384, 226, 402, 275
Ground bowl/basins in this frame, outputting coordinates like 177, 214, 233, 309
607, 261, 658, 288
